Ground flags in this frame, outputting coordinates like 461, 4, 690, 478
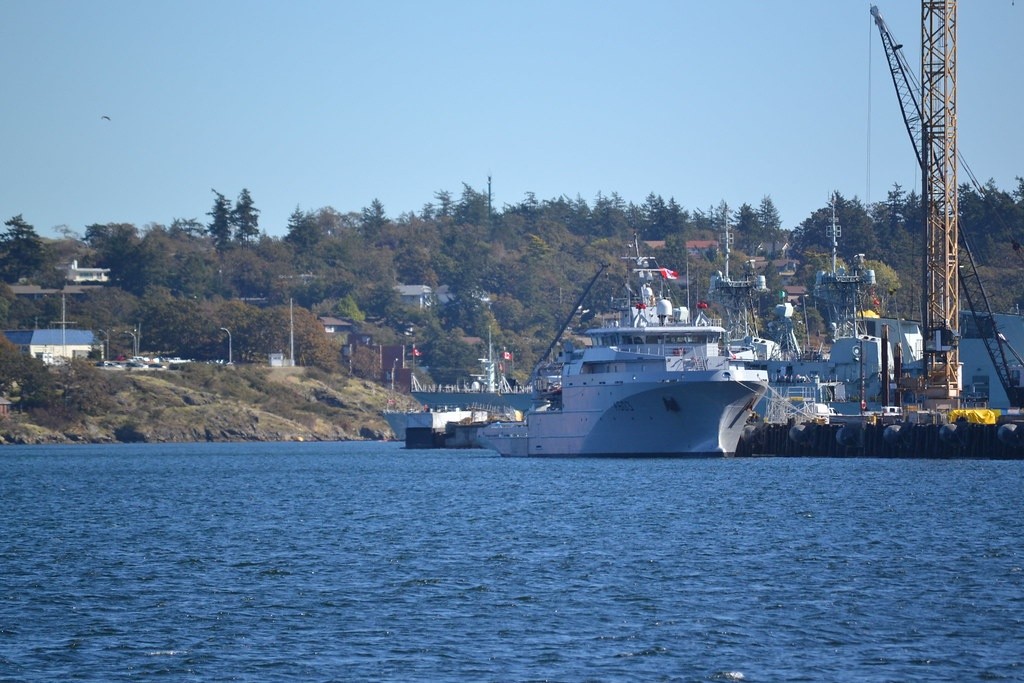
502, 350, 512, 360
414, 349, 422, 357
660, 266, 678, 280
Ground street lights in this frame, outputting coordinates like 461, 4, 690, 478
97, 328, 110, 359
221, 327, 232, 362
124, 331, 136, 356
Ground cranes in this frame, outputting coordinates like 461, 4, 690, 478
872, 0, 1023, 407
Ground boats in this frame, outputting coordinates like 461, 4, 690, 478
384, 403, 493, 441
406, 321, 553, 414
475, 229, 771, 458
